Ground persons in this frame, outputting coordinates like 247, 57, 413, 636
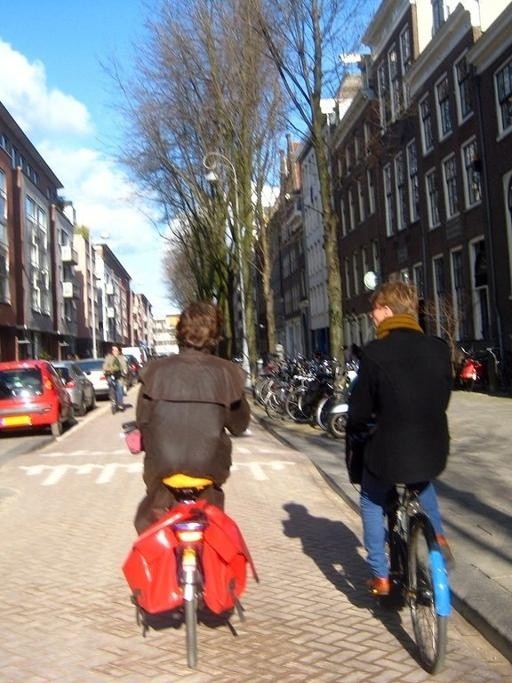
342, 278, 458, 597
130, 298, 255, 538
348, 342, 362, 360
119, 346, 130, 397
103, 343, 128, 410
340, 345, 350, 362
63, 347, 93, 360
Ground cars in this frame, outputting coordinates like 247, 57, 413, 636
0, 345, 145, 439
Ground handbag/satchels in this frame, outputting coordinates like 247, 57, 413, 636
123, 501, 250, 614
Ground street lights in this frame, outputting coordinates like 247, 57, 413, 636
203, 149, 254, 389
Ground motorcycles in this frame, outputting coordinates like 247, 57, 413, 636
455, 346, 482, 391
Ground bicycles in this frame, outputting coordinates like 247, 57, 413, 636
253, 351, 361, 440
123, 414, 248, 670
373, 482, 453, 673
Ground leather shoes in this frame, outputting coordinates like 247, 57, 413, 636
366, 576, 390, 595
438, 533, 456, 567
